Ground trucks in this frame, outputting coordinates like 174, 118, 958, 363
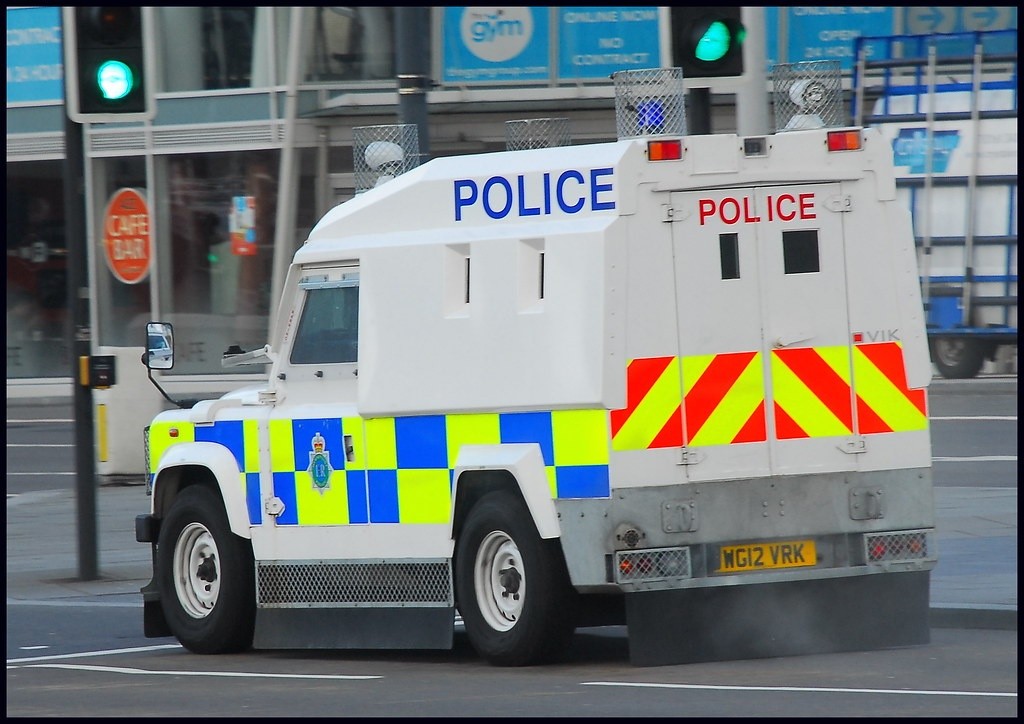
872, 88, 1017, 380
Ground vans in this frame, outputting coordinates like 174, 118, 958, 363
141, 67, 938, 668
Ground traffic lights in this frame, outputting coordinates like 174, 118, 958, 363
667, 4, 753, 86
62, 4, 157, 122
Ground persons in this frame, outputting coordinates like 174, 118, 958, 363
13, 196, 68, 346
